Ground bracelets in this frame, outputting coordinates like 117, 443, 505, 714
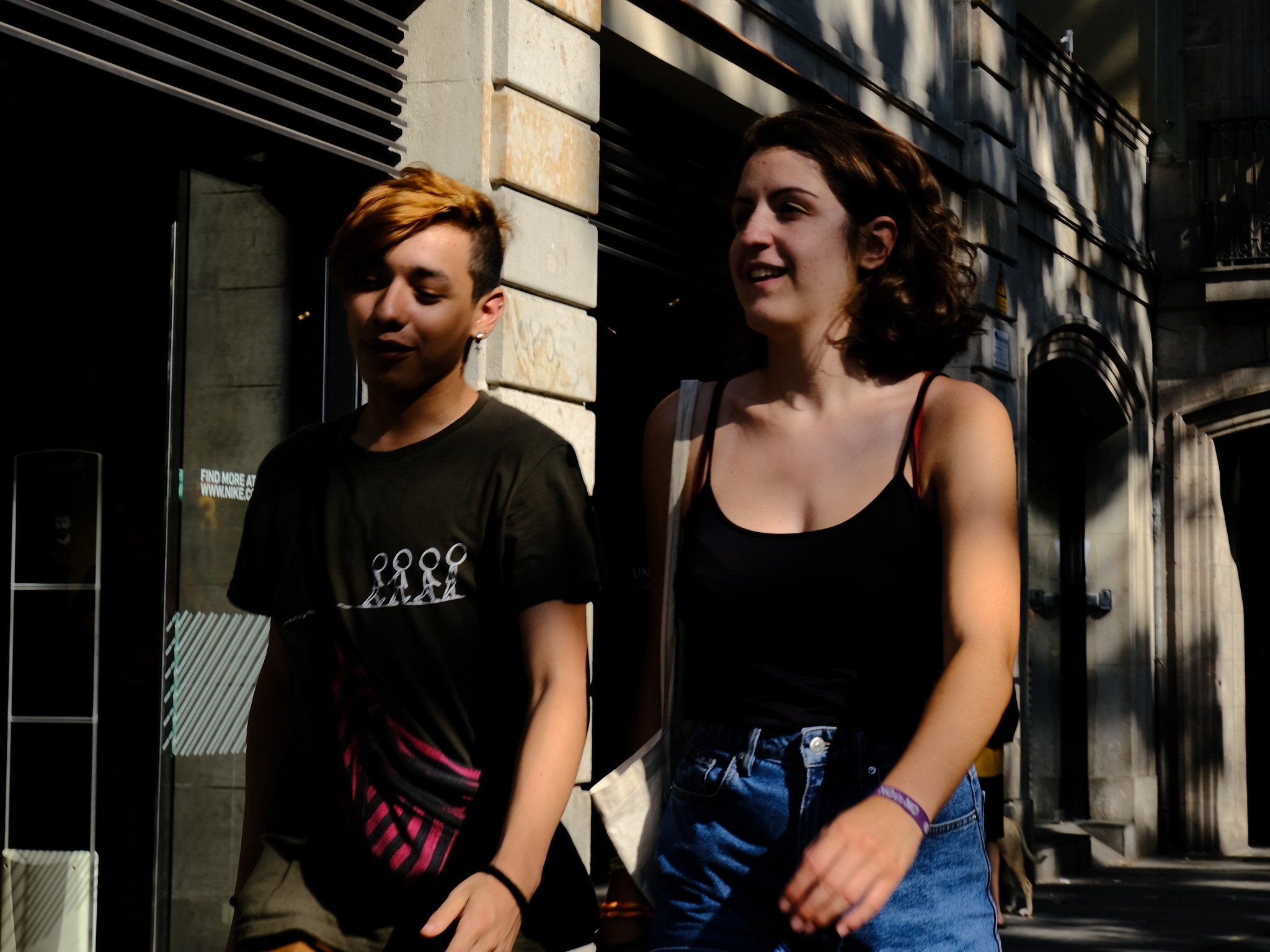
877, 784, 932, 837
481, 865, 528, 918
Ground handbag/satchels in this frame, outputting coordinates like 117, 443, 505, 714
322, 640, 485, 879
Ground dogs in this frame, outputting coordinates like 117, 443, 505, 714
987, 815, 1047, 929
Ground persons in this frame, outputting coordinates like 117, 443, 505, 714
625, 105, 1022, 952
225, 164, 599, 952
